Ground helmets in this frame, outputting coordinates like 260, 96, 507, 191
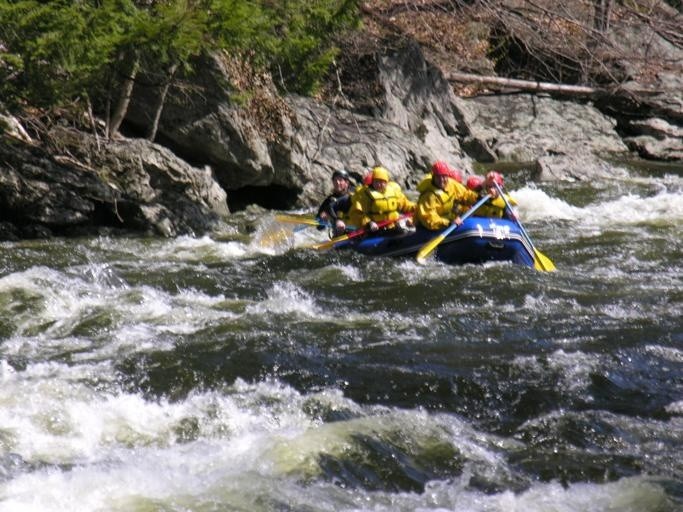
432, 161, 503, 192
332, 166, 389, 186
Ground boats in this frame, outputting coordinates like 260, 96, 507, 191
258, 212, 535, 269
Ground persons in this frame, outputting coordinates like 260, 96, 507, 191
316, 160, 518, 238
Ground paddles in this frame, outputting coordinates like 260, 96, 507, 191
418, 194, 492, 260
313, 212, 412, 252
278, 213, 359, 231
491, 175, 556, 272
